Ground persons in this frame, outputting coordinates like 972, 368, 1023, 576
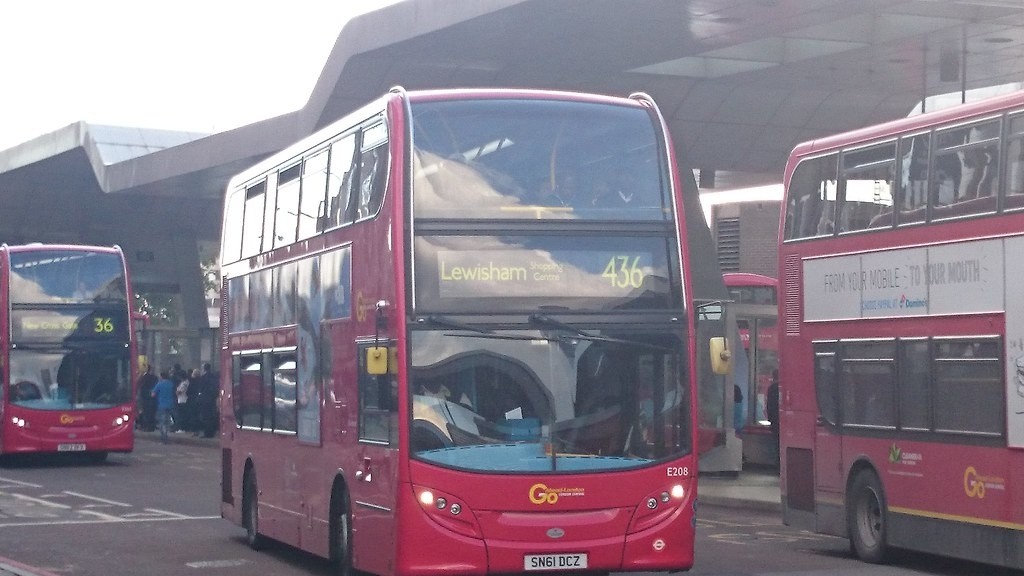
733, 384, 746, 468
132, 347, 223, 449
766, 365, 784, 468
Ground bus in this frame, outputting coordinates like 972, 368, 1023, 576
774, 82, 1024, 576
0, 242, 150, 467
216, 82, 699, 573
